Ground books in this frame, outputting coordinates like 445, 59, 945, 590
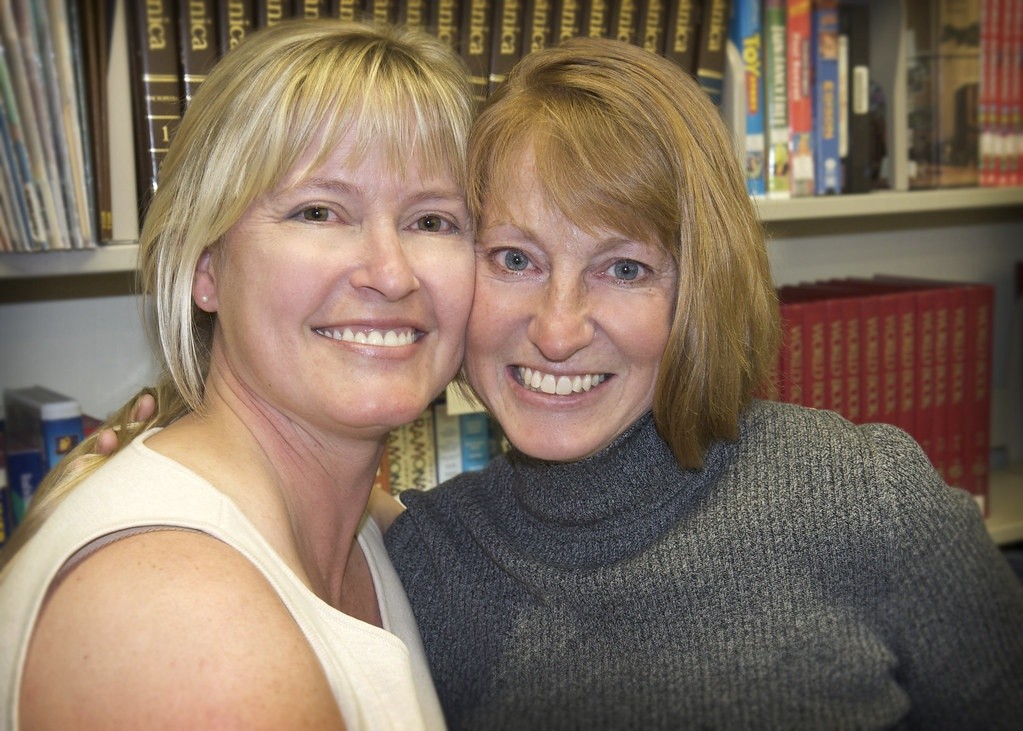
908, 0, 1023, 190
0, 383, 106, 549
373, 391, 507, 498
760, 276, 996, 521
0, 0, 886, 253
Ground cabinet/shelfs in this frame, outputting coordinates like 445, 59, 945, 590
1, 0, 1022, 554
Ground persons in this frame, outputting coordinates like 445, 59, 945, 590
0, 20, 475, 731
95, 35, 1023, 731
